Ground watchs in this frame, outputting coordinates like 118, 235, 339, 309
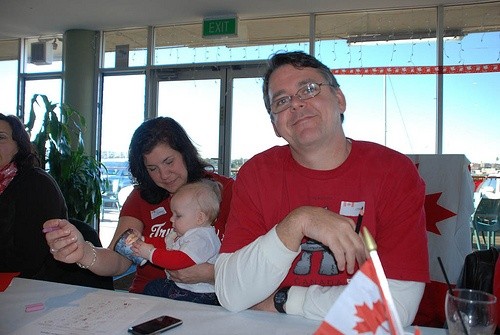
274, 286, 291, 313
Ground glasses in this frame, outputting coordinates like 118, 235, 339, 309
267, 83, 335, 114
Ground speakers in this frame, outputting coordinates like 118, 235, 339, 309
31, 42, 52, 65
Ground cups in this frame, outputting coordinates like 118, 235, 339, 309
445, 289, 497, 335
113, 229, 147, 266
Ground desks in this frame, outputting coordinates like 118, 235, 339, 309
0, 278, 449, 335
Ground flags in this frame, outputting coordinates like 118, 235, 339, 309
313, 255, 397, 335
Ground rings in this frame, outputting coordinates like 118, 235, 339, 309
50, 247, 56, 253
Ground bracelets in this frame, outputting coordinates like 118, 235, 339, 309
76, 241, 97, 269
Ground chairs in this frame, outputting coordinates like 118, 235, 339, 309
403, 154, 500, 335
100, 179, 120, 223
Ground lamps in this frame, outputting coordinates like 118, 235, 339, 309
346, 30, 465, 46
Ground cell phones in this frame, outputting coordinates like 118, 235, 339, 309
128, 316, 182, 335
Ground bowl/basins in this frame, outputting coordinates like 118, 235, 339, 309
0, 272, 20, 293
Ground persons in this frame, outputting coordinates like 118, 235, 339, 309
215, 49, 429, 328
0, 113, 67, 282
43, 116, 235, 294
130, 180, 222, 305
